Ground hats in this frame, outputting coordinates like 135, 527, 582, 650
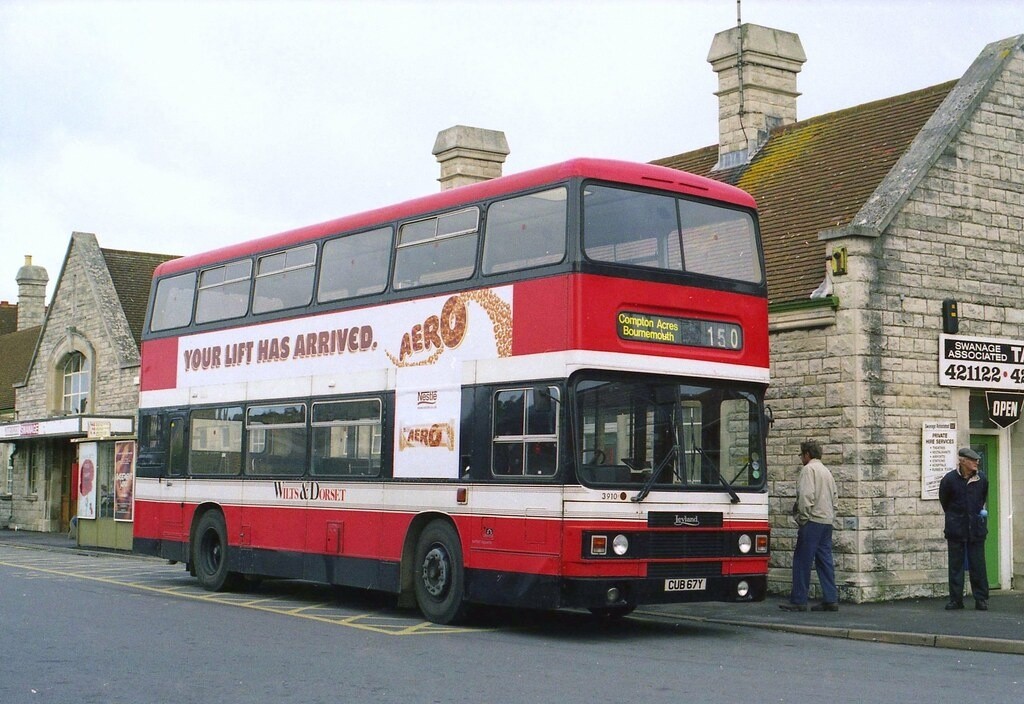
958, 448, 981, 460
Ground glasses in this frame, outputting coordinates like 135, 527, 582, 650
798, 452, 804, 460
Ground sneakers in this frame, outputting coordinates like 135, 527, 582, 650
945, 601, 963, 610
779, 600, 808, 611
976, 599, 988, 609
811, 603, 838, 611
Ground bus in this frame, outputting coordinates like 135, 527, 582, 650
132, 158, 775, 625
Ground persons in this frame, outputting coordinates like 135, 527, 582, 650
939, 448, 990, 610
779, 441, 839, 611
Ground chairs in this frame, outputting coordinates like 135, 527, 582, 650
399, 281, 426, 292
251, 457, 370, 474
